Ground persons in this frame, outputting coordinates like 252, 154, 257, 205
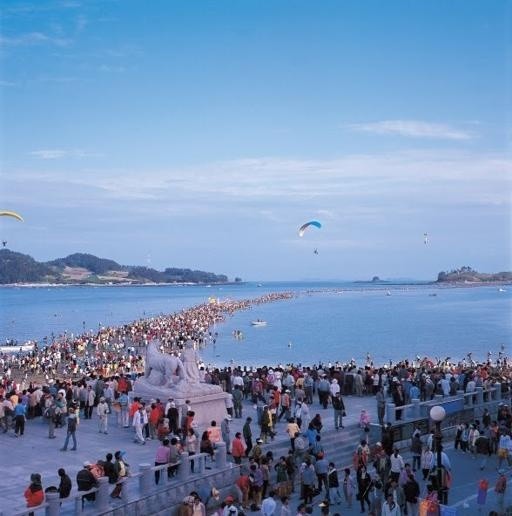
0, 346, 512, 516
6, 290, 294, 347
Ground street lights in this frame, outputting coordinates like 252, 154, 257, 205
429, 405, 447, 504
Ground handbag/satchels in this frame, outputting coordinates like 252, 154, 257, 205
365, 424, 369, 432
312, 487, 321, 497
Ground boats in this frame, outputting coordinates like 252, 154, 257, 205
1, 344, 37, 353
250, 320, 266, 325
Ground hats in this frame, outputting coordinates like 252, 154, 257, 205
31, 473, 42, 485
256, 438, 264, 443
212, 487, 220, 501
225, 414, 232, 421
285, 389, 291, 393
498, 469, 504, 476
69, 408, 74, 413
376, 442, 381, 447
100, 397, 106, 401
83, 461, 89, 467
